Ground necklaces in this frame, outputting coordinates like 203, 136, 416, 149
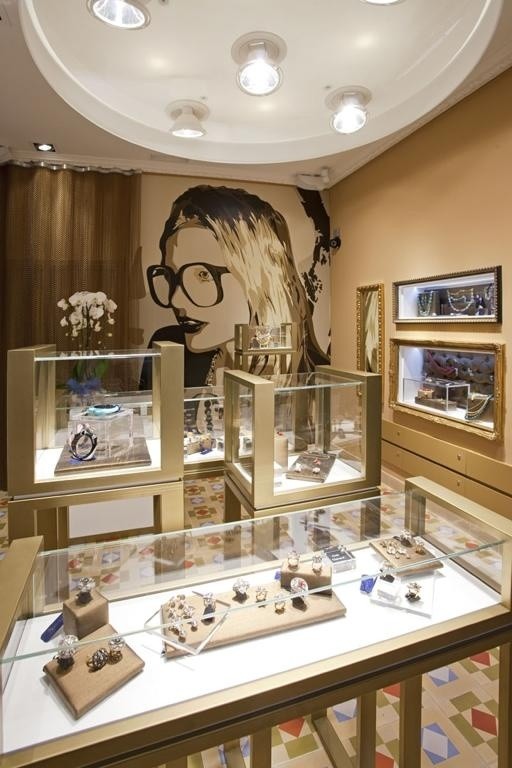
204, 353, 219, 433
482, 286, 493, 300
462, 392, 495, 420
303, 453, 331, 461
415, 290, 434, 317
446, 287, 474, 312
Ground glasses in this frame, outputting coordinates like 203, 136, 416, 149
145, 260, 233, 308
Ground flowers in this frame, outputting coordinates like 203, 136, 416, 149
55, 286, 117, 379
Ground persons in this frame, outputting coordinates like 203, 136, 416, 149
135, 183, 331, 454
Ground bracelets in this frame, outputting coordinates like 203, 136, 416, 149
87, 401, 121, 416
69, 430, 99, 462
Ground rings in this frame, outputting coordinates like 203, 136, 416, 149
87, 648, 110, 668
108, 633, 125, 647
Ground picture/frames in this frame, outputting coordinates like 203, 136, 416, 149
355, 282, 385, 412
